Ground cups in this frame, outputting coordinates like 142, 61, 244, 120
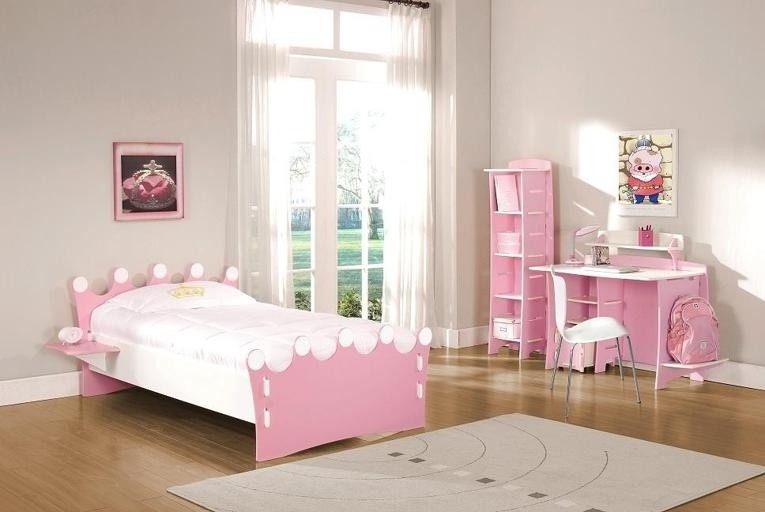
639, 230, 654, 246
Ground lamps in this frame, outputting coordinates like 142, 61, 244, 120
562, 224, 601, 267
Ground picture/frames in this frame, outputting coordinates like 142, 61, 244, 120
111, 141, 185, 223
612, 127, 681, 220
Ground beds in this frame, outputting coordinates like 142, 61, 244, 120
41, 260, 435, 460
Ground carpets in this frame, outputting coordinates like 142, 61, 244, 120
165, 409, 765, 511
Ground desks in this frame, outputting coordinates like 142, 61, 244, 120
527, 253, 732, 392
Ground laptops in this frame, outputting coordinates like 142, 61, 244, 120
583, 264, 639, 273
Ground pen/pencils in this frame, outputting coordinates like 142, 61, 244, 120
639, 224, 652, 231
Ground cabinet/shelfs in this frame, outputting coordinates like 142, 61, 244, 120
481, 157, 556, 363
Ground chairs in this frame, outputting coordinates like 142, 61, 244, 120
549, 264, 642, 422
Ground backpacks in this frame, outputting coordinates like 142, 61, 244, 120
666, 294, 720, 365
